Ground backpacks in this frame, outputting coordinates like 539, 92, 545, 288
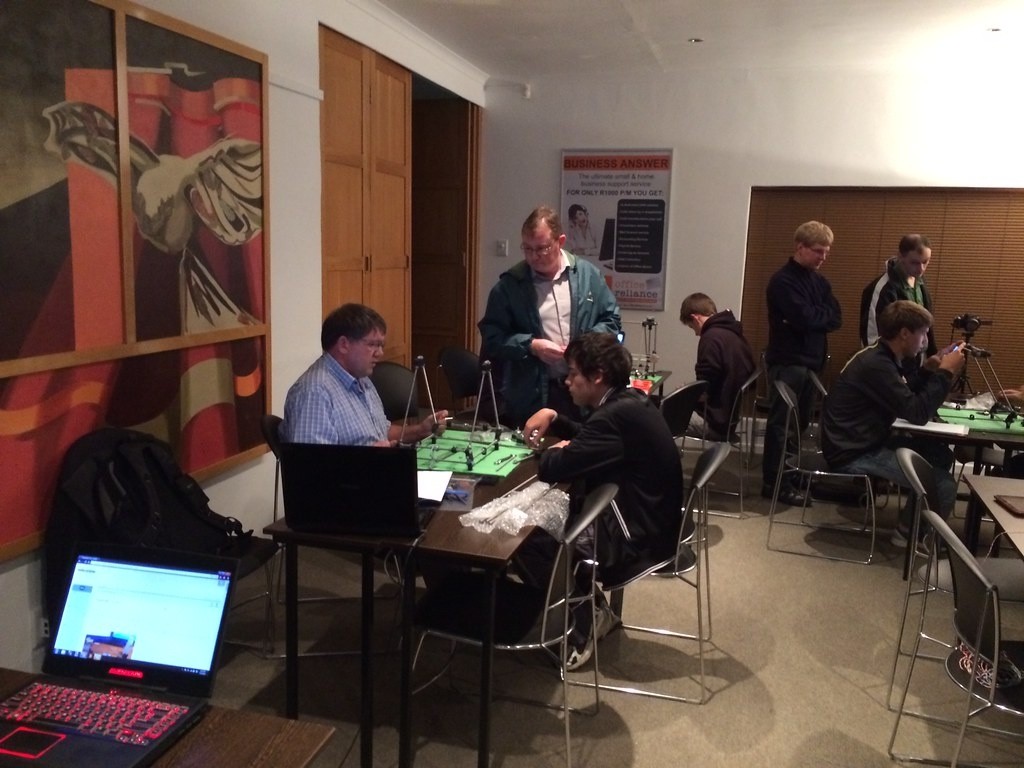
99, 431, 254, 564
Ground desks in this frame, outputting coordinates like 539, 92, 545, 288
627, 369, 673, 410
889, 390, 1024, 557
264, 420, 574, 768
965, 473, 1024, 561
0, 667, 338, 768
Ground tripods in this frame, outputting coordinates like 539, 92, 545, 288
951, 332, 975, 395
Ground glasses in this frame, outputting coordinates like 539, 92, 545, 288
358, 337, 386, 352
520, 240, 554, 256
806, 244, 830, 256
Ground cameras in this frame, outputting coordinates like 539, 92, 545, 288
954, 314, 992, 333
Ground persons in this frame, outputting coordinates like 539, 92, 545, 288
477, 207, 621, 440
761, 220, 843, 507
821, 300, 965, 558
282, 304, 448, 448
568, 204, 602, 256
986, 385, 1024, 480
520, 330, 683, 673
648, 292, 757, 444
859, 234, 939, 380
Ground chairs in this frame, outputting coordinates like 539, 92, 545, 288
38, 338, 1024, 768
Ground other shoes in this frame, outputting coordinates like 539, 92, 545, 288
763, 469, 816, 490
761, 483, 813, 506
890, 524, 947, 553
953, 444, 975, 464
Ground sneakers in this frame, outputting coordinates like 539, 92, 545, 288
590, 591, 622, 642
562, 588, 612, 670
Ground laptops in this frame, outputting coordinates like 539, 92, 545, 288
0, 542, 241, 768
277, 441, 436, 539
